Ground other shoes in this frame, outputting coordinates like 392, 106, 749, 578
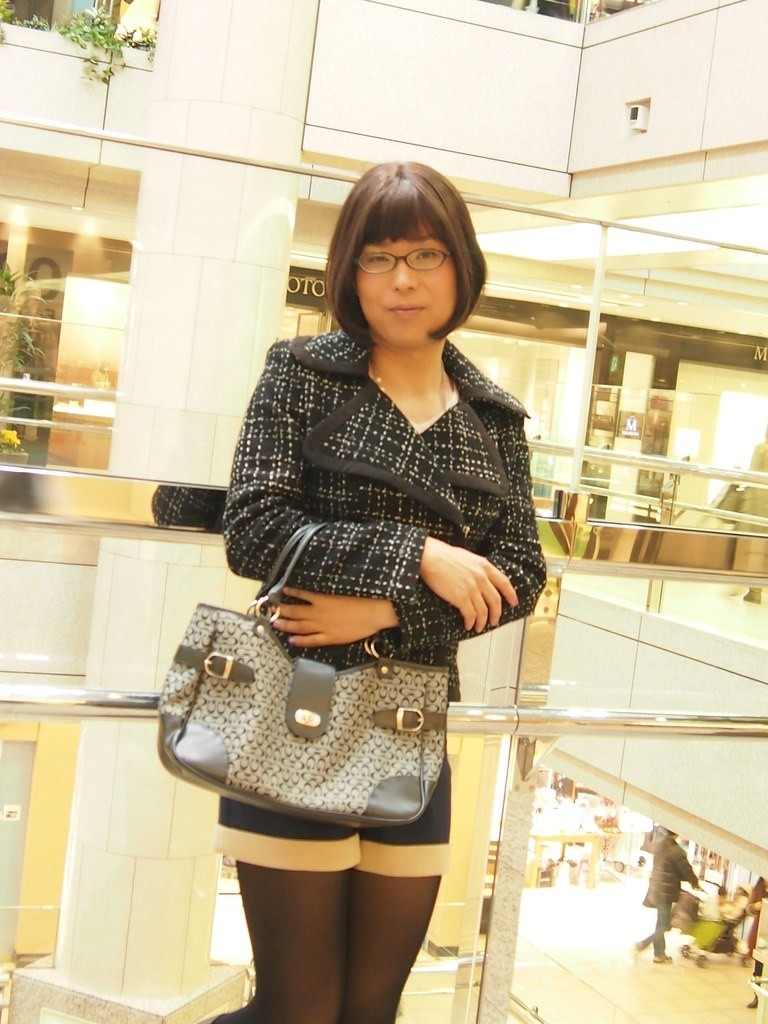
747, 996, 760, 1008
633, 941, 643, 952
680, 944, 691, 959
697, 954, 707, 969
654, 954, 672, 964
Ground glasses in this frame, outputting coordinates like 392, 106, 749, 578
351, 245, 452, 271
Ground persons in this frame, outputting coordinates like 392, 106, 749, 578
194, 158, 549, 1024
744, 877, 768, 1008
732, 427, 768, 534
633, 825, 702, 964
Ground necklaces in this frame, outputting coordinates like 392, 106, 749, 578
371, 362, 385, 393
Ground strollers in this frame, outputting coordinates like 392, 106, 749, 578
670, 878, 752, 967
594, 816, 646, 872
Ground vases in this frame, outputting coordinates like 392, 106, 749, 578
0, 450, 27, 464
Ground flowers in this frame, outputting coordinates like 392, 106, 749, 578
0, 261, 46, 454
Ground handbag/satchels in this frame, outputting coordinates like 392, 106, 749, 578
156, 525, 449, 824
643, 877, 657, 908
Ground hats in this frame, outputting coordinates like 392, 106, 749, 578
738, 882, 752, 896
